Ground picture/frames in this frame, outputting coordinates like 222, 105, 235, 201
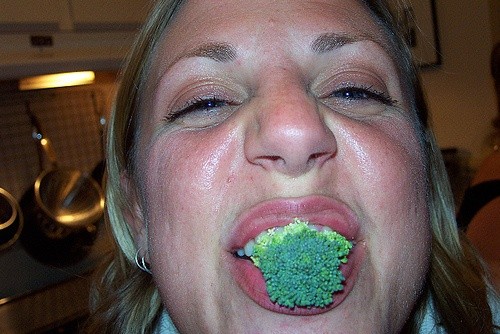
389, 0, 443, 71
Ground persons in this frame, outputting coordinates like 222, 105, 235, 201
87, 0, 500, 333
454, 44, 500, 294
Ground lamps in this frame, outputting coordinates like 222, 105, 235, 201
18, 70, 96, 91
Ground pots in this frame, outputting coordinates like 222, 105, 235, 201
23, 93, 106, 240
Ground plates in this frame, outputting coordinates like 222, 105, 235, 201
0, 188, 24, 253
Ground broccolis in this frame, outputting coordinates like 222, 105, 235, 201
250, 216, 353, 310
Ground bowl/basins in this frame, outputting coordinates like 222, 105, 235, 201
0, 194, 17, 231
